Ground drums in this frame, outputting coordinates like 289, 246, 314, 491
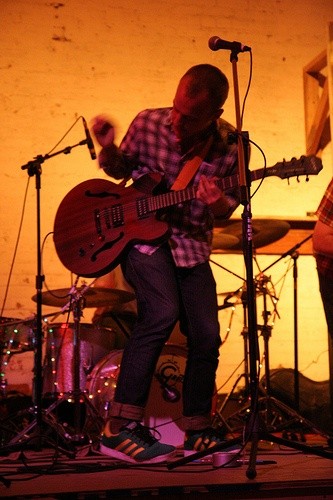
92, 308, 137, 344
83, 344, 217, 448
40, 322, 118, 403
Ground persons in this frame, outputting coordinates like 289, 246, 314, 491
313, 176, 333, 447
89, 64, 251, 464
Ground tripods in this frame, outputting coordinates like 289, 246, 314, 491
0, 50, 333, 486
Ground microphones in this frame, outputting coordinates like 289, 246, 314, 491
208, 35, 250, 52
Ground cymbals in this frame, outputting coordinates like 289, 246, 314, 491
212, 218, 290, 250
30, 284, 136, 309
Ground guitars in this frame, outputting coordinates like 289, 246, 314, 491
52, 153, 324, 279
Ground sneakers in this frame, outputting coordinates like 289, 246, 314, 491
184, 429, 245, 462
100, 421, 178, 465
